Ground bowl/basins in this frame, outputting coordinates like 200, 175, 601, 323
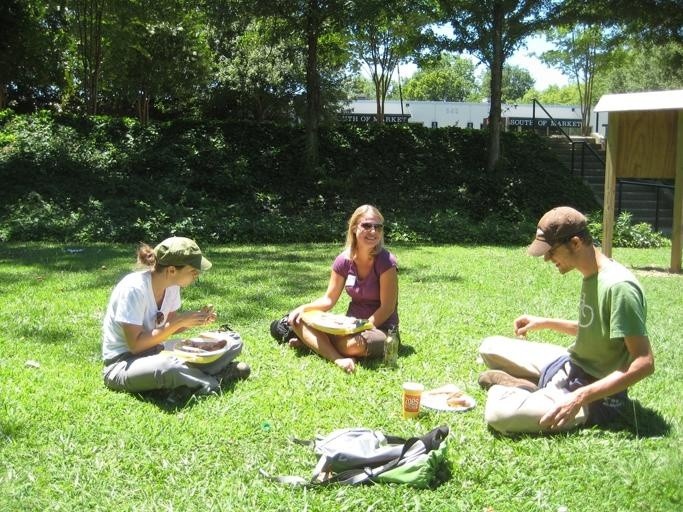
173, 331, 233, 365
302, 309, 371, 337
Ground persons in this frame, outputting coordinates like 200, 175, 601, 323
474, 202, 657, 441
98, 234, 251, 405
266, 202, 404, 377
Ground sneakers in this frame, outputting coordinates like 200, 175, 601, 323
215, 361, 251, 385
478, 369, 539, 392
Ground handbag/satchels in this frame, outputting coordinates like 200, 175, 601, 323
270, 314, 297, 344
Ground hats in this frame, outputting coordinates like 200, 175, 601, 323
154, 236, 213, 272
526, 206, 587, 257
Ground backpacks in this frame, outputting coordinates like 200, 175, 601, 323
310, 425, 451, 489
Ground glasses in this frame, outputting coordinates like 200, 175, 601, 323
156, 310, 165, 325
356, 222, 385, 232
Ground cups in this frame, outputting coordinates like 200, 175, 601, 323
401, 380, 426, 416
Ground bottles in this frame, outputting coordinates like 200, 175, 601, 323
383, 323, 399, 366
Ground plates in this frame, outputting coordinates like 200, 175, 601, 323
417, 389, 478, 413
